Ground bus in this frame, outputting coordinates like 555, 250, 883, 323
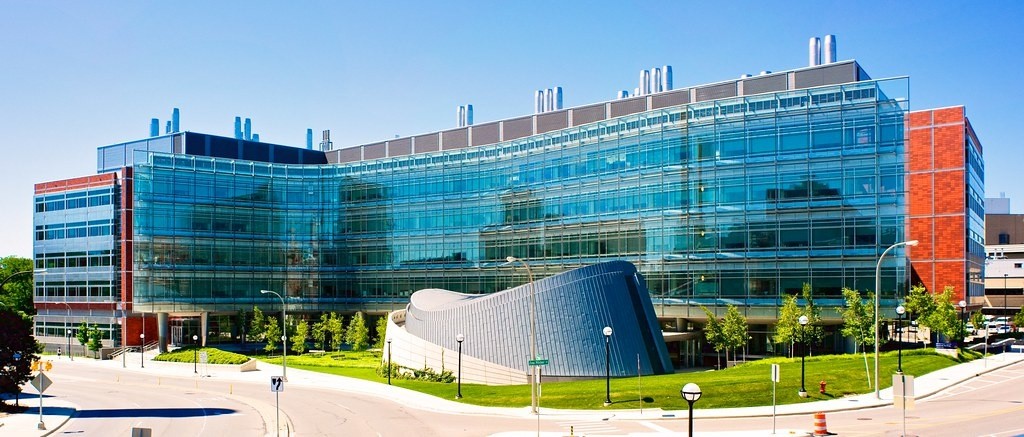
981, 306, 1022, 330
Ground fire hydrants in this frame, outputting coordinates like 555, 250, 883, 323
819, 380, 827, 395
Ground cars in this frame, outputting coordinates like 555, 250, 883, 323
967, 315, 1012, 334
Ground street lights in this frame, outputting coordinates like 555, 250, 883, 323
894, 305, 906, 378
505, 256, 539, 413
456, 333, 466, 399
874, 241, 919, 398
958, 300, 968, 357
680, 383, 702, 436
798, 314, 809, 397
261, 290, 290, 385
56, 301, 76, 362
140, 334, 146, 367
601, 327, 615, 406
67, 329, 77, 361
1004, 273, 1008, 334
387, 337, 393, 385
193, 334, 199, 375
